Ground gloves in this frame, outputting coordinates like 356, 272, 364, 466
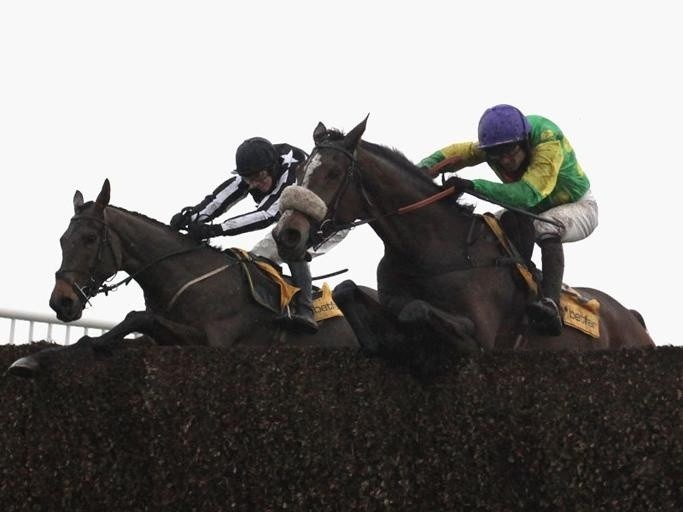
441, 175, 474, 201
172, 210, 222, 243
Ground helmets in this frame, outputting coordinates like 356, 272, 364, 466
232, 137, 281, 181
477, 103, 533, 159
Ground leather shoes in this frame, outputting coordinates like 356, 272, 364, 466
530, 294, 564, 337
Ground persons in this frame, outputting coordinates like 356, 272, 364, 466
170, 134, 352, 336
403, 103, 600, 336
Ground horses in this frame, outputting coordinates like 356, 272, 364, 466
6, 177, 382, 377
271, 111, 657, 380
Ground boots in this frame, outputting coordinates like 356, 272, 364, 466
287, 272, 320, 336
